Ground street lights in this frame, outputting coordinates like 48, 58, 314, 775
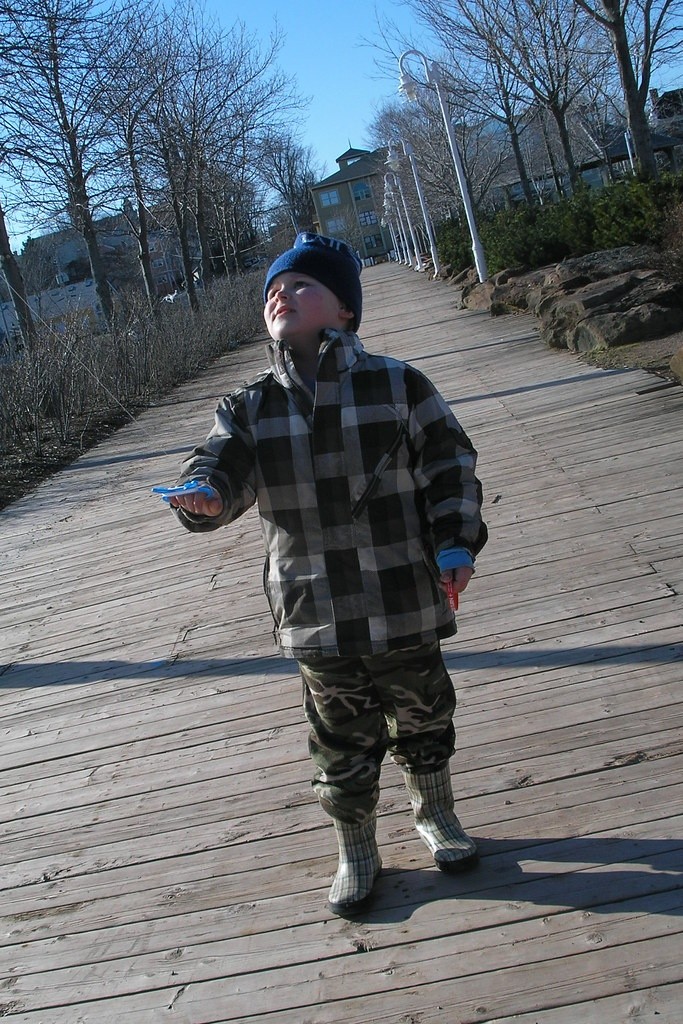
383, 171, 427, 271
384, 136, 443, 279
397, 48, 490, 283
378, 192, 415, 267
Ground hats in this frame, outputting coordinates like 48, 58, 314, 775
263, 231, 363, 333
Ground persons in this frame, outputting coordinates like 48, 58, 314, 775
169, 232, 488, 916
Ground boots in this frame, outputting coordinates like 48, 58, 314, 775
401, 762, 478, 871
328, 807, 382, 914
